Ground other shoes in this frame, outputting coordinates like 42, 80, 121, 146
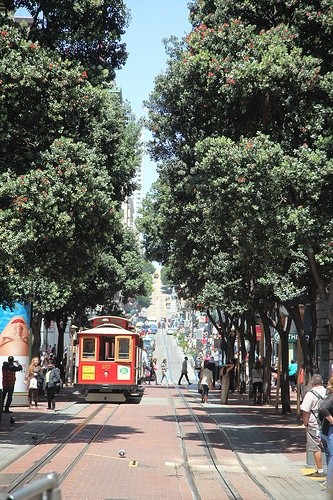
4, 407, 13, 413
178, 383, 182, 385
189, 383, 192, 385
307, 471, 325, 477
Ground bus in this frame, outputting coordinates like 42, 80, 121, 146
73, 316, 144, 404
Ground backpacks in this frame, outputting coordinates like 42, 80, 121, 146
307, 390, 324, 426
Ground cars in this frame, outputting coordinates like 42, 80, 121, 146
167, 328, 174, 335
137, 323, 158, 381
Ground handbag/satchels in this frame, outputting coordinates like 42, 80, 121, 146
198, 381, 203, 394
29, 378, 37, 388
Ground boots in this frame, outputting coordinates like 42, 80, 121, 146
202, 394, 211, 404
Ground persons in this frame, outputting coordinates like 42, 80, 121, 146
178, 356, 192, 385
160, 359, 169, 385
200, 363, 215, 404
152, 358, 158, 370
148, 358, 161, 385
40, 343, 69, 410
1, 356, 23, 412
177, 319, 333, 495
24, 357, 43, 408
